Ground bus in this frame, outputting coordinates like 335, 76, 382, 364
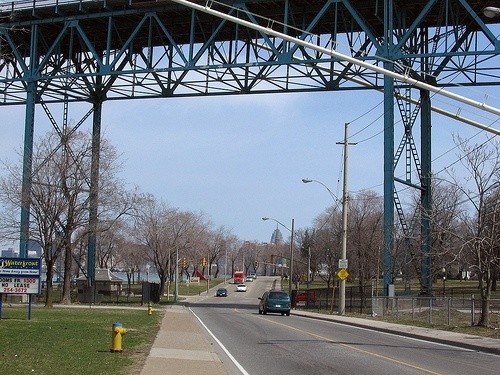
233, 270, 245, 284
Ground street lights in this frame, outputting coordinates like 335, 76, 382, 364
302, 178, 348, 316
262, 217, 295, 298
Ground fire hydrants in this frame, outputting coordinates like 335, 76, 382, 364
110, 320, 129, 353
147, 303, 155, 317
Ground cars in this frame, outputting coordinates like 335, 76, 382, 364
258, 289, 292, 316
295, 290, 318, 304
246, 276, 253, 282
250, 273, 257, 279
236, 283, 248, 292
216, 287, 228, 297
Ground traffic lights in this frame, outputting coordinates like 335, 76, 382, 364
182, 257, 188, 270
202, 258, 208, 268
255, 261, 259, 268
